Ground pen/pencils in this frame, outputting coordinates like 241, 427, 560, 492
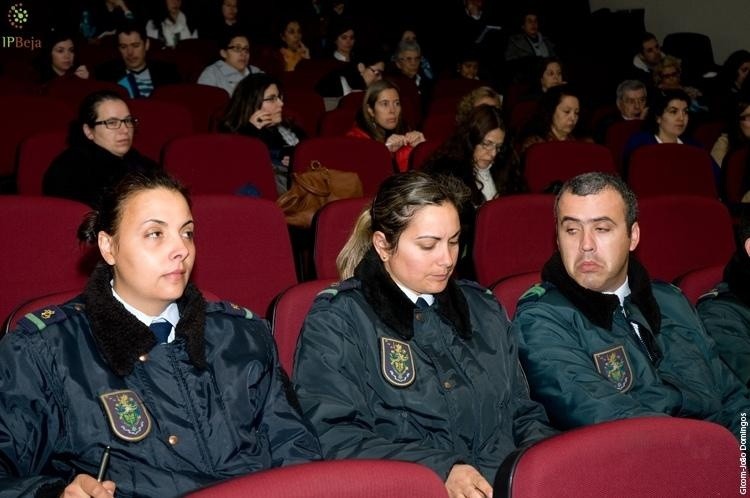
91, 445, 110, 498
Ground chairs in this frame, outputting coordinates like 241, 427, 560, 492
1, 0, 747, 498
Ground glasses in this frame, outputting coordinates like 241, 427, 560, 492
367, 65, 383, 77
263, 93, 286, 104
224, 45, 252, 54
91, 115, 140, 130
395, 56, 422, 62
481, 140, 503, 151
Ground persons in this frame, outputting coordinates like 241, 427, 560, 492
289, 166, 565, 498
696, 214, 750, 386
419, 103, 530, 282
512, 170, 750, 465
0, 162, 324, 496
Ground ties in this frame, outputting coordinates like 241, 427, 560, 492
147, 321, 173, 346
414, 297, 431, 310
